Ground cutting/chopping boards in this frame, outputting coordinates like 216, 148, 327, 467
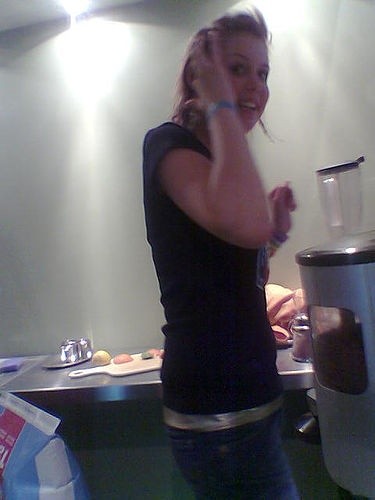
68, 349, 164, 377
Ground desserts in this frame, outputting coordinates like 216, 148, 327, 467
92, 348, 165, 365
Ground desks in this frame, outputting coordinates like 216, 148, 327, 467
0, 341, 317, 408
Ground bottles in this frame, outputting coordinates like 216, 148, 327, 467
291, 312, 313, 364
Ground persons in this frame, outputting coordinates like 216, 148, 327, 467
140, 5, 304, 500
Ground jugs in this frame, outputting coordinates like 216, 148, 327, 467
316, 162, 364, 240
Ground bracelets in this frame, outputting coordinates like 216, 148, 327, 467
204, 100, 235, 120
263, 226, 289, 256
